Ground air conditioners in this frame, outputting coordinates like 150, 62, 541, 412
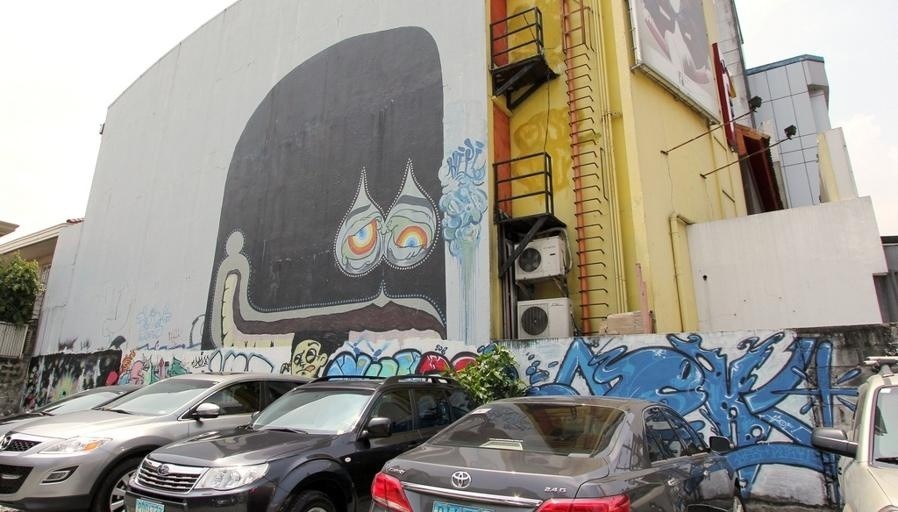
514, 236, 574, 341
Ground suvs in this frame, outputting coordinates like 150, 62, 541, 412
811, 356, 898, 512
